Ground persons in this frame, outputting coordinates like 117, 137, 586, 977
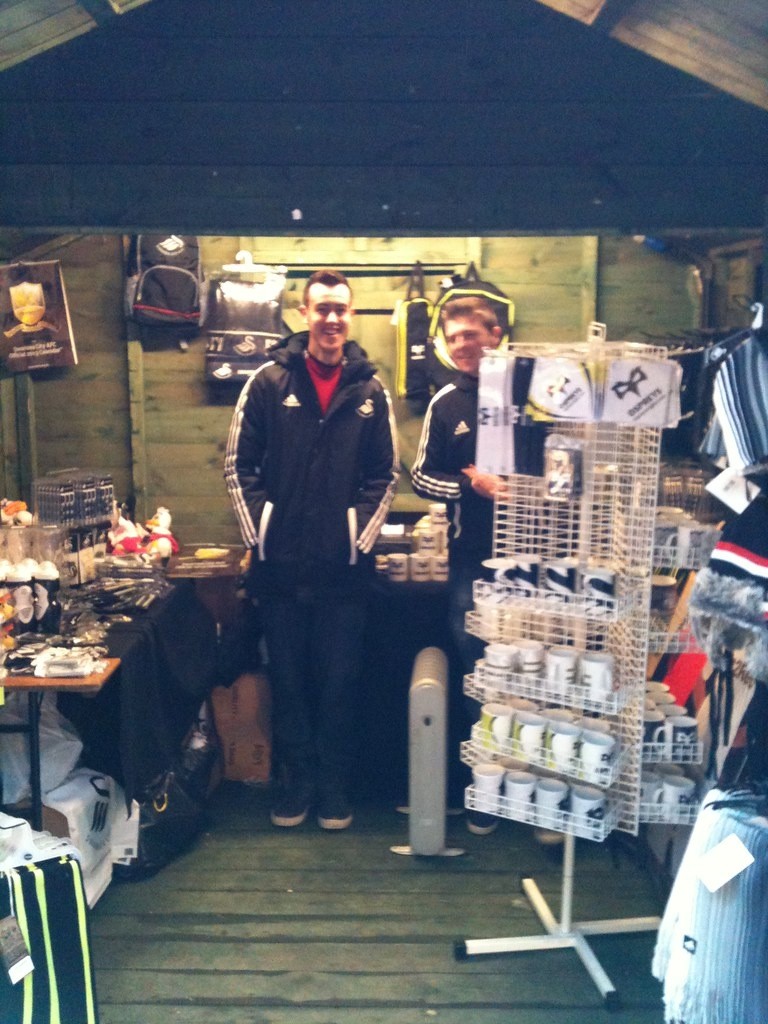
409, 297, 565, 843
223, 272, 400, 829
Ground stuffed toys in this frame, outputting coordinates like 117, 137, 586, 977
109, 504, 177, 567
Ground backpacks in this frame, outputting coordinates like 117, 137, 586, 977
431, 261, 516, 390
205, 278, 283, 398
121, 233, 208, 340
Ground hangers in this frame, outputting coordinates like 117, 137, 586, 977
223, 250, 287, 275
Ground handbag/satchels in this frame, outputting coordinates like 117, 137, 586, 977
113, 687, 224, 886
394, 296, 434, 400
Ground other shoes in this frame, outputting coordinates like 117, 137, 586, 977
271, 796, 311, 827
466, 812, 500, 835
395, 800, 466, 815
318, 800, 354, 830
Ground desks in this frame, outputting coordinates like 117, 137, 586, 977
0, 581, 175, 831
166, 543, 247, 643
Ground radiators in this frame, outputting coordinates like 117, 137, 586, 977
390, 646, 467, 858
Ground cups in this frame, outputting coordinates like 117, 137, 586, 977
480, 703, 514, 752
542, 709, 573, 723
513, 640, 544, 686
640, 764, 697, 822
481, 556, 517, 604
575, 717, 611, 734
571, 786, 606, 836
547, 648, 578, 695
633, 680, 696, 760
544, 561, 578, 603
621, 568, 678, 621
377, 504, 449, 582
508, 699, 540, 713
532, 768, 563, 784
472, 765, 505, 810
579, 733, 615, 784
535, 781, 569, 828
484, 645, 514, 688
511, 556, 541, 598
545, 721, 581, 773
580, 656, 615, 704
544, 434, 581, 500
499, 758, 530, 783
504, 769, 536, 820
512, 710, 547, 762
568, 778, 604, 792
654, 505, 722, 568
580, 568, 616, 619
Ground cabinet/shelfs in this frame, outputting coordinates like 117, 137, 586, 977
454, 339, 726, 1014
